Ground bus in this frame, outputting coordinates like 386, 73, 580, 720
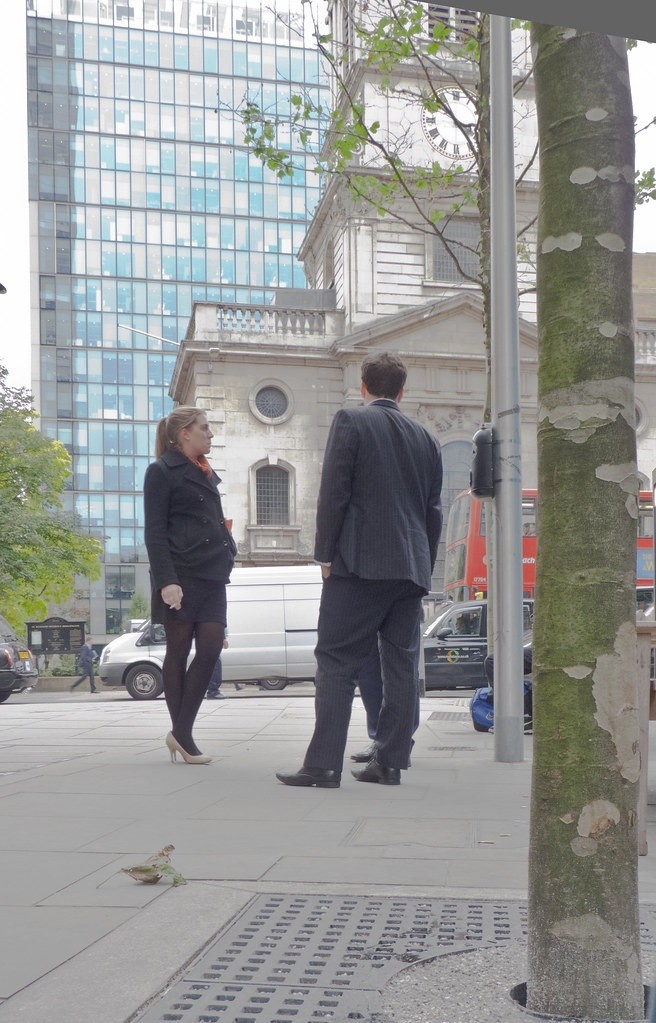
443, 482, 656, 601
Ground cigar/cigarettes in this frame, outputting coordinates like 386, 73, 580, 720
170, 605, 175, 609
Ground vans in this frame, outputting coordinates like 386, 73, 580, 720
97, 564, 324, 702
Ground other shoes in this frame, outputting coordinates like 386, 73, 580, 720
488, 714, 533, 734
207, 692, 227, 699
91, 691, 99, 693
70, 685, 74, 692
351, 742, 376, 761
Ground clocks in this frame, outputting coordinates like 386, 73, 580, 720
422, 84, 480, 161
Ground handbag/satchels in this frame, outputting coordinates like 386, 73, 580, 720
470, 685, 495, 731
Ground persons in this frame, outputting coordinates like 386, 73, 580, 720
484, 644, 534, 733
143, 406, 237, 764
451, 617, 471, 636
69, 637, 100, 693
276, 352, 443, 790
204, 627, 229, 699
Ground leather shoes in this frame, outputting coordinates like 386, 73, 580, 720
276, 765, 342, 787
351, 757, 401, 785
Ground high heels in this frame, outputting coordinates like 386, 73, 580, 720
166, 731, 212, 763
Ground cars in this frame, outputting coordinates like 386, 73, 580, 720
0, 614, 39, 704
424, 592, 533, 691
